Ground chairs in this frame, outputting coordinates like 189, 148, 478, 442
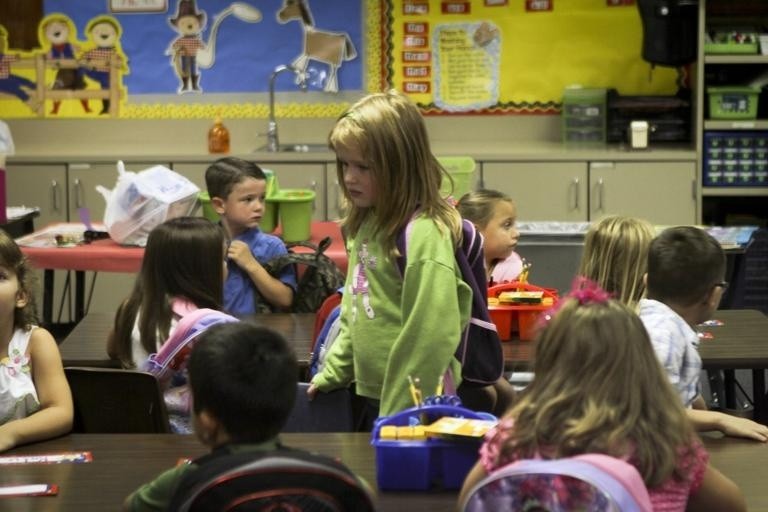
63, 367, 170, 433
275, 382, 354, 433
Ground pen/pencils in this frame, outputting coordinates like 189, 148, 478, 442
405, 375, 424, 408
436, 374, 445, 397
516, 259, 532, 292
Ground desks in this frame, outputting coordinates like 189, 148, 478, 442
516, 220, 761, 296
0, 430, 768, 512
59, 310, 768, 423
15, 221, 348, 330
6, 206, 39, 238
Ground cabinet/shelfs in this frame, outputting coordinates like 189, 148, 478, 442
6, 163, 171, 324
696, 0, 768, 224
172, 164, 325, 223
481, 161, 696, 224
325, 161, 481, 221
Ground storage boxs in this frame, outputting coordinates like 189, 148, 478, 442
707, 87, 761, 120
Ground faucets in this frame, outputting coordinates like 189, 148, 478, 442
256, 63, 308, 152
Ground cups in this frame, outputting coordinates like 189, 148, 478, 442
629, 120, 649, 149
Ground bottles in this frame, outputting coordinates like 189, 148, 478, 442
206, 111, 230, 154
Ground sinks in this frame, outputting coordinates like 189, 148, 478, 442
253, 142, 335, 154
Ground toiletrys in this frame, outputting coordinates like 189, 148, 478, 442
209, 104, 229, 154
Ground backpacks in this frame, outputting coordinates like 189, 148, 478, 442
255, 236, 346, 312
461, 416, 653, 512
168, 443, 375, 512
395, 197, 505, 387
309, 287, 344, 378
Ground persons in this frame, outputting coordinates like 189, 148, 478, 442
204, 157, 297, 317
122, 323, 379, 510
571, 214, 652, 308
635, 226, 768, 443
457, 274, 748, 510
1, 227, 74, 452
450, 190, 520, 413
0, 117, 15, 224
106, 216, 237, 434
305, 89, 474, 431
